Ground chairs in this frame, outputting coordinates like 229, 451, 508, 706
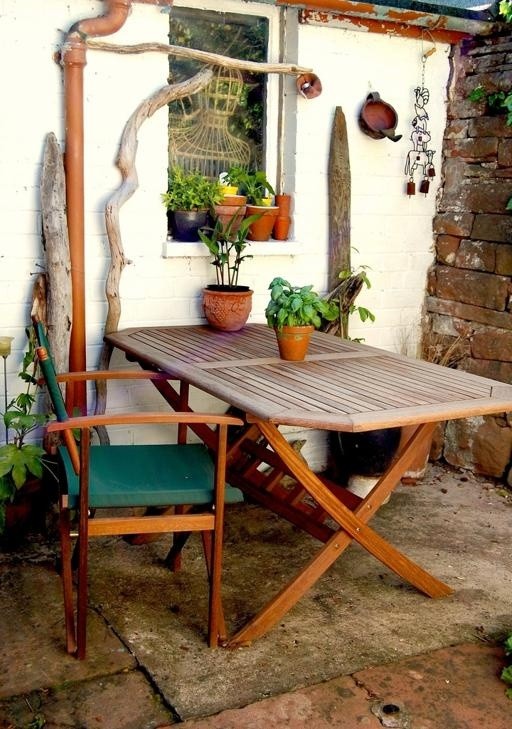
28, 297, 247, 664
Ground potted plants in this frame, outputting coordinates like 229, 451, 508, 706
193, 201, 267, 333
162, 165, 278, 242
264, 276, 331, 361
0, 325, 82, 555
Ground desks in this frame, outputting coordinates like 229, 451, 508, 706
101, 320, 512, 648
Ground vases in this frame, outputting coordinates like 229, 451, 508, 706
272, 194, 292, 239
335, 423, 433, 506
